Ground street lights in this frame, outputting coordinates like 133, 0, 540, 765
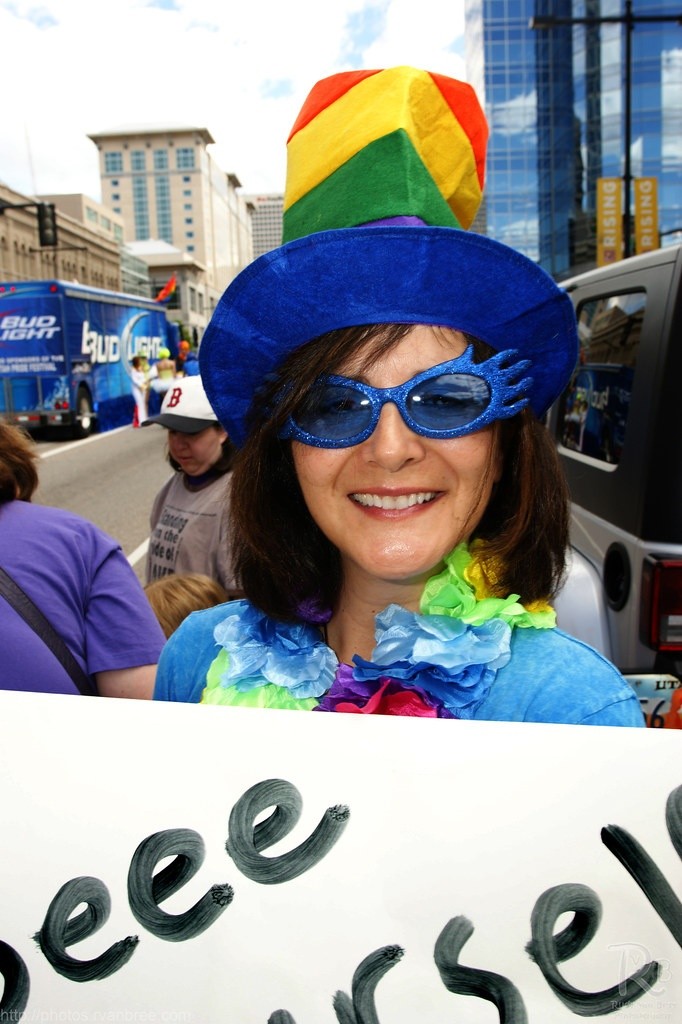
527, 0, 681, 259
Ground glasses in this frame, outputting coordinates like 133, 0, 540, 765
269, 344, 537, 447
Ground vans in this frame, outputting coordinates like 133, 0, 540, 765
533, 246, 682, 677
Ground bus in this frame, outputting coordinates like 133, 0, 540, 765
0, 279, 185, 438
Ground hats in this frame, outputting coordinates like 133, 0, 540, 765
195, 224, 580, 445
144, 376, 221, 432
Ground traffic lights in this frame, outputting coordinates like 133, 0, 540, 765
38, 204, 56, 246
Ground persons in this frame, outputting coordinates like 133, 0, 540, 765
132, 338, 204, 430
0, 418, 168, 701
562, 397, 590, 451
149, 371, 246, 592
141, 569, 228, 640
145, 64, 650, 732
